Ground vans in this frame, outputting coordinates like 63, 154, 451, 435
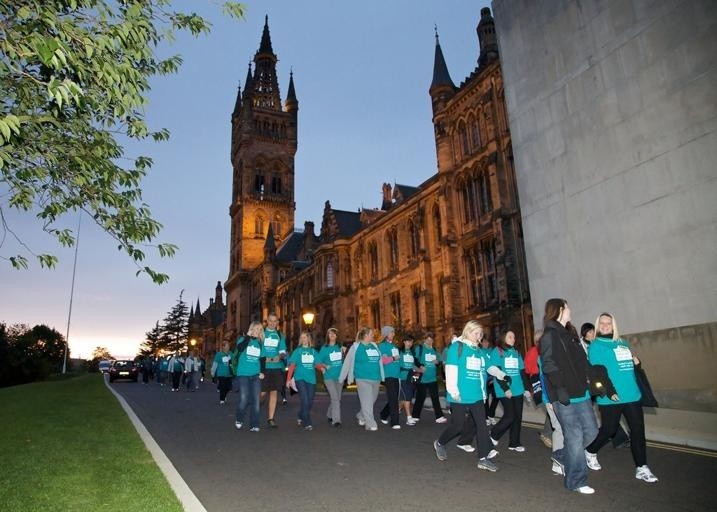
97, 360, 110, 374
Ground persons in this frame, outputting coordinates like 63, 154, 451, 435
587, 310, 660, 484
140, 349, 206, 393
211, 313, 630, 475
537, 298, 602, 494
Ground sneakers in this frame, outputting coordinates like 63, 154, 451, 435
434, 417, 658, 494
236, 417, 417, 432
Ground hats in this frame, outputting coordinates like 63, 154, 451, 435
382, 326, 394, 337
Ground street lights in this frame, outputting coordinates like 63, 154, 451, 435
189, 338, 197, 351
61, 190, 87, 375
298, 308, 316, 337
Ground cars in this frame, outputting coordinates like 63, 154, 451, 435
108, 360, 137, 384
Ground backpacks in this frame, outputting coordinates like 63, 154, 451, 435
232, 337, 262, 376
